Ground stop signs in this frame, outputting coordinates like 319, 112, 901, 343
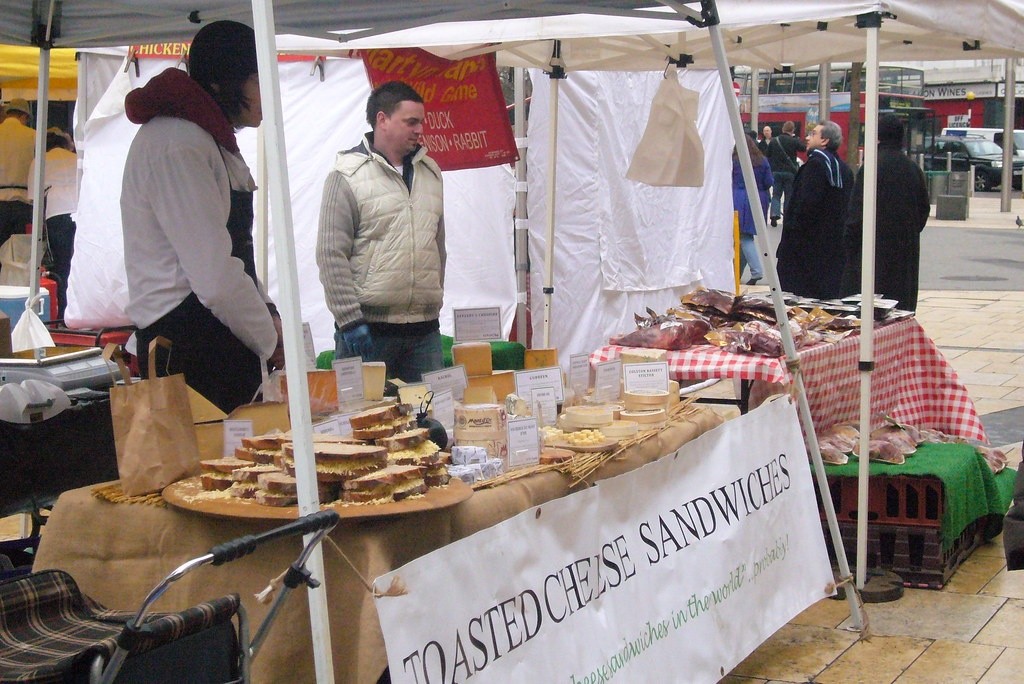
733, 81, 740, 97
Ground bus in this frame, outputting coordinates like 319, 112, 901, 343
732, 65, 926, 180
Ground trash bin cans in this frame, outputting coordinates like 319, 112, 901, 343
922, 170, 971, 206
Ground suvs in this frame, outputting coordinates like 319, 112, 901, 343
924, 137, 1024, 192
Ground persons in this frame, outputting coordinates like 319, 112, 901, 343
838, 110, 930, 318
118, 19, 285, 415
26, 124, 78, 329
748, 130, 759, 146
731, 133, 773, 285
315, 82, 448, 399
0, 98, 36, 272
767, 120, 807, 227
757, 125, 774, 159
774, 119, 855, 299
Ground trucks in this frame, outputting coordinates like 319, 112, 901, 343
941, 127, 1024, 158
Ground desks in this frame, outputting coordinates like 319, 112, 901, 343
44, 380, 742, 684
590, 290, 984, 444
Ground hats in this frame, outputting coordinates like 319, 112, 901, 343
4, 98, 34, 122
47, 127, 75, 152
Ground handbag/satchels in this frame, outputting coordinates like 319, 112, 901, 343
102, 336, 202, 496
792, 162, 800, 175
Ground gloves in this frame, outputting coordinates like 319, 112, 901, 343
342, 324, 375, 361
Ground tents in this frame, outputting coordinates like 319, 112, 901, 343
0, 1, 1023, 684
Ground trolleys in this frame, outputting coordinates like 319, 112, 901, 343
0, 507, 339, 684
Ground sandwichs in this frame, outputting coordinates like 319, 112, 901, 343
199, 403, 448, 509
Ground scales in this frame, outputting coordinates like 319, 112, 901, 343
0, 346, 121, 392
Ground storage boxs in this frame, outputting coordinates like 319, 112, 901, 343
783, 437, 993, 592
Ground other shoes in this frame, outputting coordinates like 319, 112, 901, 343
746, 277, 762, 285
771, 218, 777, 227
